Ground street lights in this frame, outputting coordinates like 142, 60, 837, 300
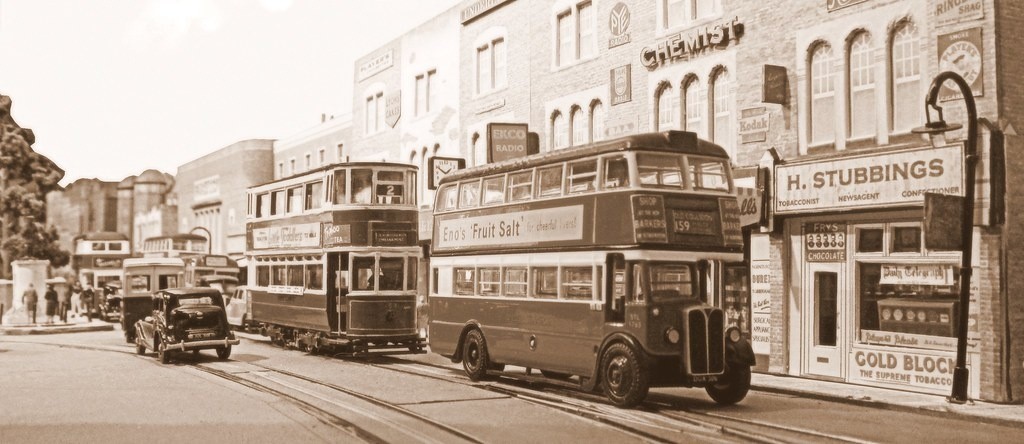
910, 68, 979, 405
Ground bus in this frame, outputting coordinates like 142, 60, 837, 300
138, 232, 208, 257
244, 162, 428, 358
120, 256, 188, 343
426, 128, 756, 408
185, 253, 241, 305
54, 232, 132, 279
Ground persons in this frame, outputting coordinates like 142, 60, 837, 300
22, 282, 38, 323
71, 280, 83, 318
45, 286, 60, 323
60, 284, 73, 322
81, 282, 94, 322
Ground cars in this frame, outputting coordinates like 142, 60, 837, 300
224, 283, 266, 332
131, 286, 241, 365
77, 268, 126, 325
